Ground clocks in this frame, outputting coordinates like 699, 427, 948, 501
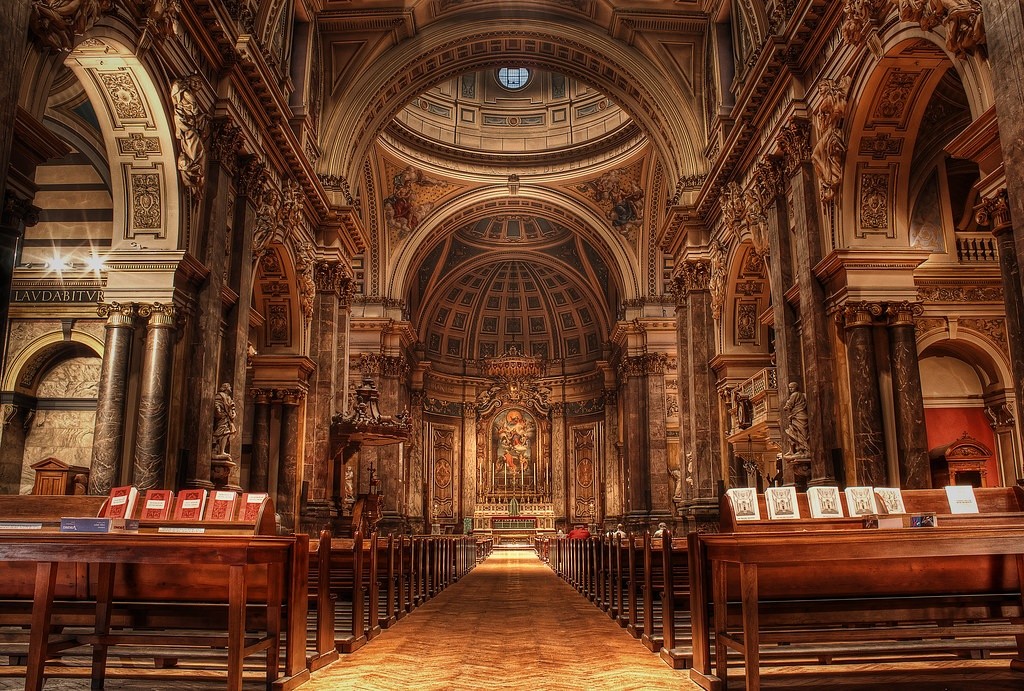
737, 395, 752, 429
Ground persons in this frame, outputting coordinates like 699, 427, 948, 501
344, 465, 354, 499
343, 397, 412, 430
214, 383, 236, 462
558, 522, 672, 540
783, 382, 811, 459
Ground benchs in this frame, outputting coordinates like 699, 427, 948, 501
533, 486, 1024, 691
0, 494, 493, 691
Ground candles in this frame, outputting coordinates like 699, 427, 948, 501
480, 463, 482, 485
534, 463, 536, 485
546, 463, 548, 485
493, 463, 494, 485
504, 463, 506, 485
522, 462, 523, 485
